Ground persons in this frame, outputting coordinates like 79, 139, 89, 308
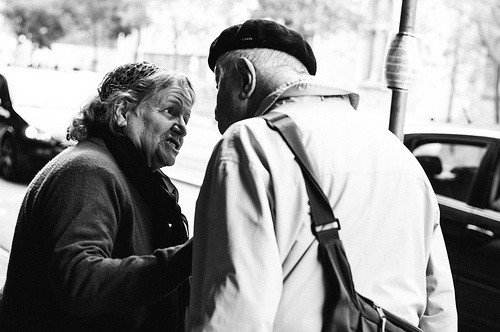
184, 19, 459, 332
0, 62, 195, 332
0, 75, 17, 177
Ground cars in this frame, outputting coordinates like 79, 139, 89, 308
0, 74, 79, 181
401, 124, 500, 329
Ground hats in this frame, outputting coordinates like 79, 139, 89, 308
208, 19, 317, 77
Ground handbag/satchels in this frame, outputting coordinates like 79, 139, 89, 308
321, 289, 423, 332
